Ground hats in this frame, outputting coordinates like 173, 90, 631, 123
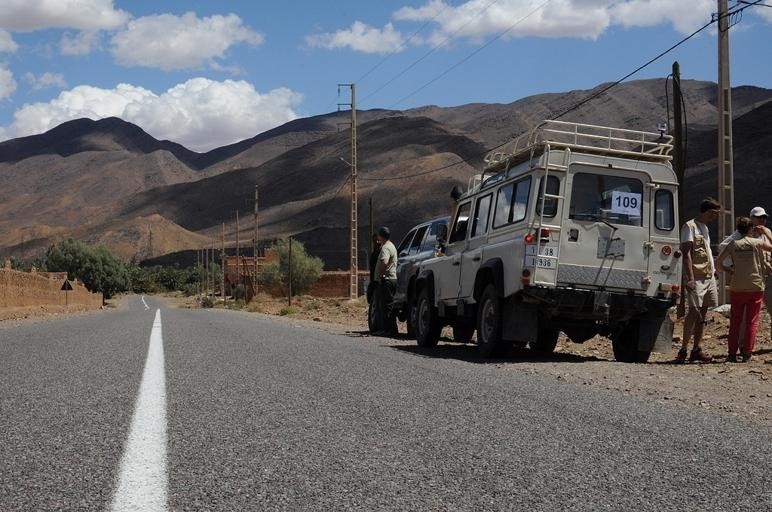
750, 206, 769, 217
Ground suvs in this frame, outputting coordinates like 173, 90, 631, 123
367, 214, 479, 336
413, 117, 681, 365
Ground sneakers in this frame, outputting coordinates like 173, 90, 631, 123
690, 350, 713, 361
743, 355, 759, 363
678, 350, 687, 360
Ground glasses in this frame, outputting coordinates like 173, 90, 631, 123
753, 215, 768, 219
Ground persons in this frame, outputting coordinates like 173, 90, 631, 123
718, 205, 771, 357
372, 225, 400, 336
673, 196, 722, 365
709, 216, 772, 365
368, 232, 383, 301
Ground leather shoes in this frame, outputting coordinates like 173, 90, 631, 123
372, 329, 383, 336
377, 331, 393, 337
